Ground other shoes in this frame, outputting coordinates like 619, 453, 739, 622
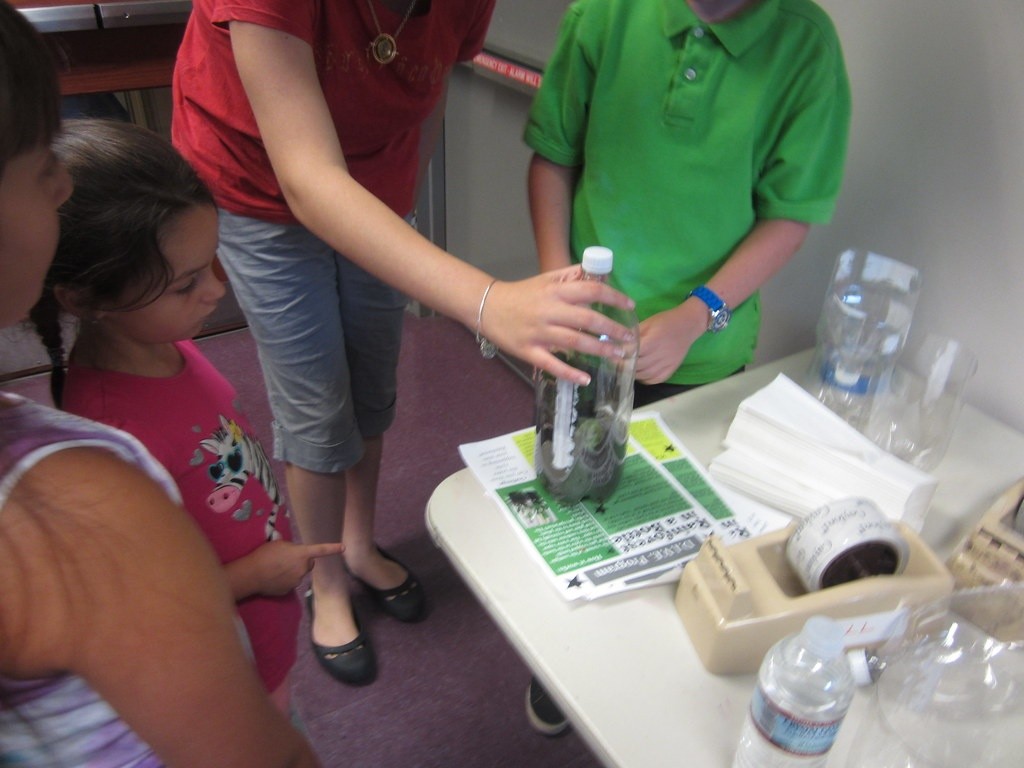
342, 541, 425, 623
524, 676, 570, 735
306, 587, 377, 684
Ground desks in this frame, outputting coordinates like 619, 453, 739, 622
427, 344, 1023, 767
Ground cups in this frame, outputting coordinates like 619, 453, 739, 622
807, 246, 921, 422
847, 582, 1024, 768
868, 329, 980, 471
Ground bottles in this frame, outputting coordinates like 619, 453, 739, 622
732, 615, 852, 768
533, 246, 640, 506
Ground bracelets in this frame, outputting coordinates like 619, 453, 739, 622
475, 279, 499, 361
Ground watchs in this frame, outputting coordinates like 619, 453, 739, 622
685, 286, 732, 333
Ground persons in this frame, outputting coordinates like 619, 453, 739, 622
171, 0, 636, 681
0, 0, 320, 768
522, 0, 852, 735
29, 117, 346, 695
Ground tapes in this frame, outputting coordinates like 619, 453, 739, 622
781, 496, 911, 596
